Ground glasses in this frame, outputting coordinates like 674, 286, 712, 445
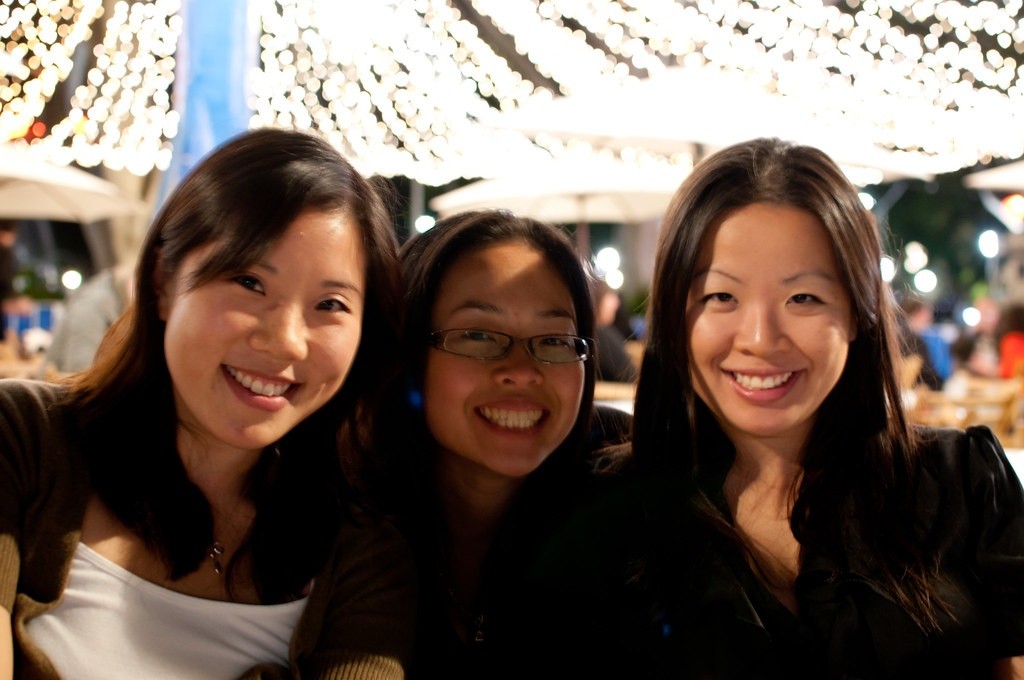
427, 327, 591, 364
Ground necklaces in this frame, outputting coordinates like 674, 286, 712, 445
208, 496, 240, 575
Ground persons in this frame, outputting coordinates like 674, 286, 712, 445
529, 139, 1024, 680
0, 122, 416, 680
882, 227, 1024, 387
587, 265, 650, 381
382, 209, 639, 679
0, 221, 139, 377
